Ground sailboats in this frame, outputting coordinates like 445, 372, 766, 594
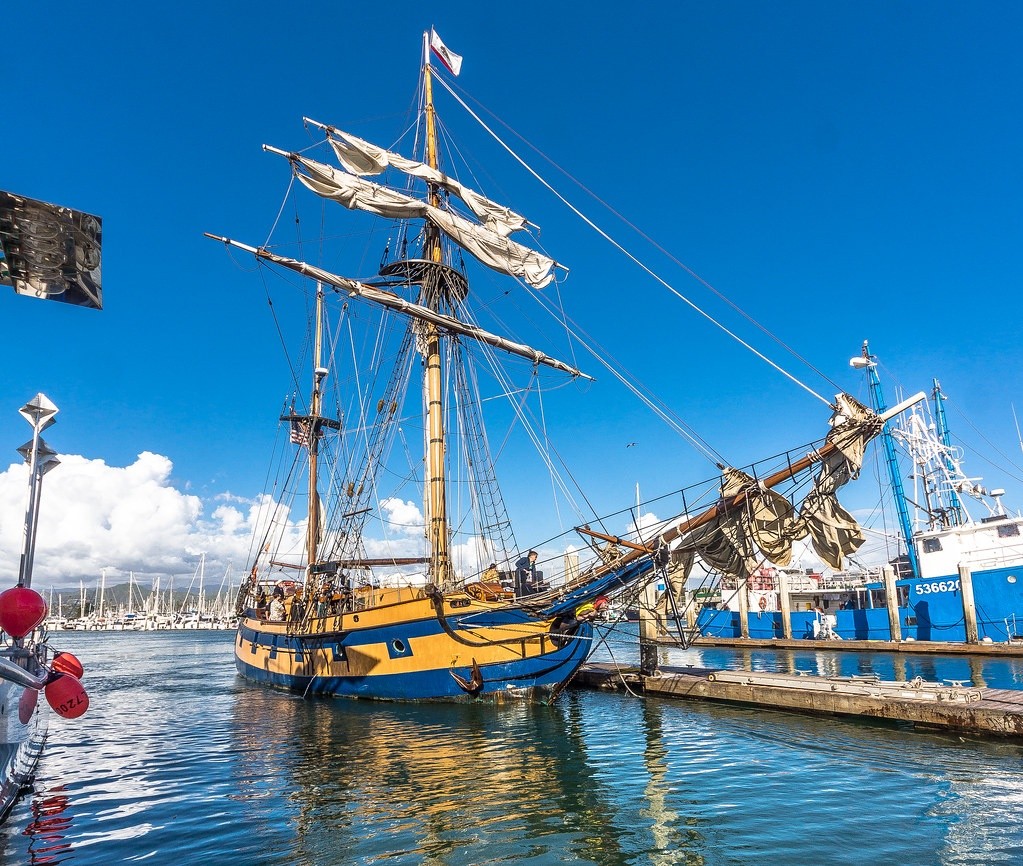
198, 21, 929, 714
31, 551, 247, 630
549, 555, 723, 623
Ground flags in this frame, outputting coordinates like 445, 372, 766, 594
430, 29, 463, 77
289, 411, 323, 447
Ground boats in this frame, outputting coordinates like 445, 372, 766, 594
690, 339, 1022, 640
0, 186, 105, 825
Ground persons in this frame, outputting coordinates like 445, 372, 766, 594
264, 578, 305, 623
480, 564, 499, 583
515, 551, 539, 596
